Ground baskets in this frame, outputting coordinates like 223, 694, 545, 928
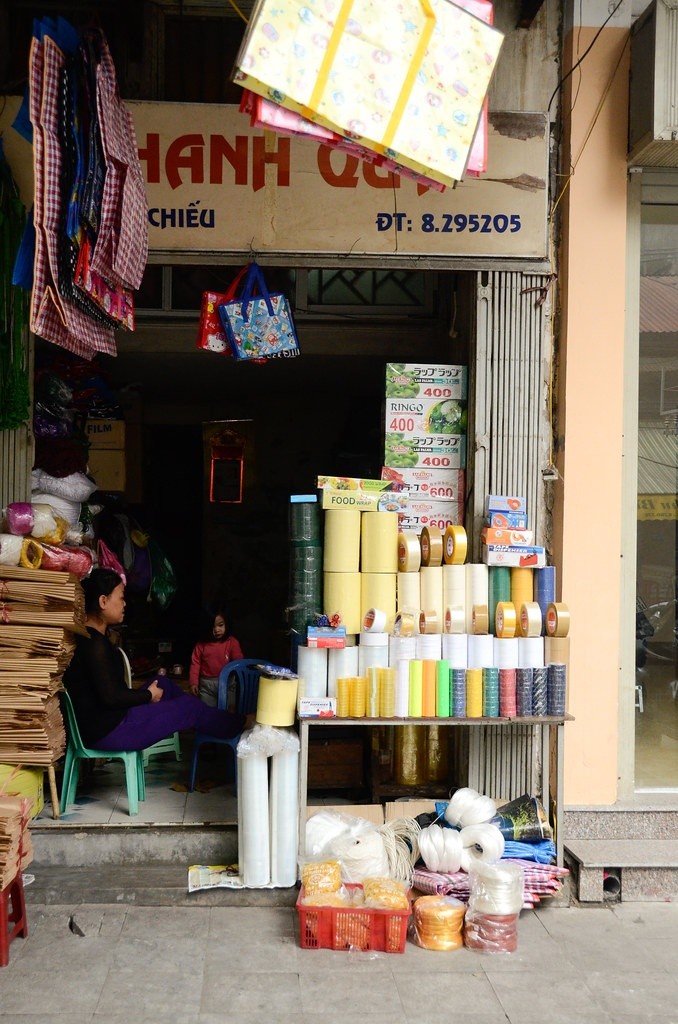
295, 884, 412, 953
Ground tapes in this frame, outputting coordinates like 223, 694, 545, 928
336, 666, 395, 717
545, 602, 570, 637
323, 508, 398, 635
472, 603, 488, 635
520, 601, 542, 637
398, 533, 421, 572
419, 610, 438, 634
360, 608, 389, 647
419, 526, 443, 567
290, 502, 321, 627
393, 612, 414, 637
255, 675, 299, 727
444, 606, 464, 633
449, 664, 566, 718
495, 601, 516, 638
444, 525, 467, 565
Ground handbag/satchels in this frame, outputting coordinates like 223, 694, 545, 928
230, 0, 504, 193
10, 15, 147, 361
196, 260, 300, 362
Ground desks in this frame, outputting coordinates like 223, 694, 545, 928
297, 711, 575, 883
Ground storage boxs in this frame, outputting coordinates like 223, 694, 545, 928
87, 450, 126, 491
308, 625, 346, 638
0, 764, 44, 818
486, 493, 526, 513
85, 419, 126, 450
386, 363, 468, 400
398, 502, 464, 536
316, 475, 394, 493
481, 527, 533, 546
384, 398, 468, 434
298, 696, 337, 718
307, 804, 385, 825
485, 511, 528, 531
384, 432, 467, 469
385, 796, 510, 822
320, 488, 410, 513
482, 544, 546, 569
381, 467, 465, 503
308, 637, 346, 649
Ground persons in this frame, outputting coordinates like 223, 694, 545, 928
189, 603, 246, 715
62, 568, 256, 753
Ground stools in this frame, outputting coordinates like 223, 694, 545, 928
141, 732, 183, 767
0, 868, 28, 967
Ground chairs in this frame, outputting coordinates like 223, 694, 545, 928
58, 689, 146, 817
189, 659, 274, 793
